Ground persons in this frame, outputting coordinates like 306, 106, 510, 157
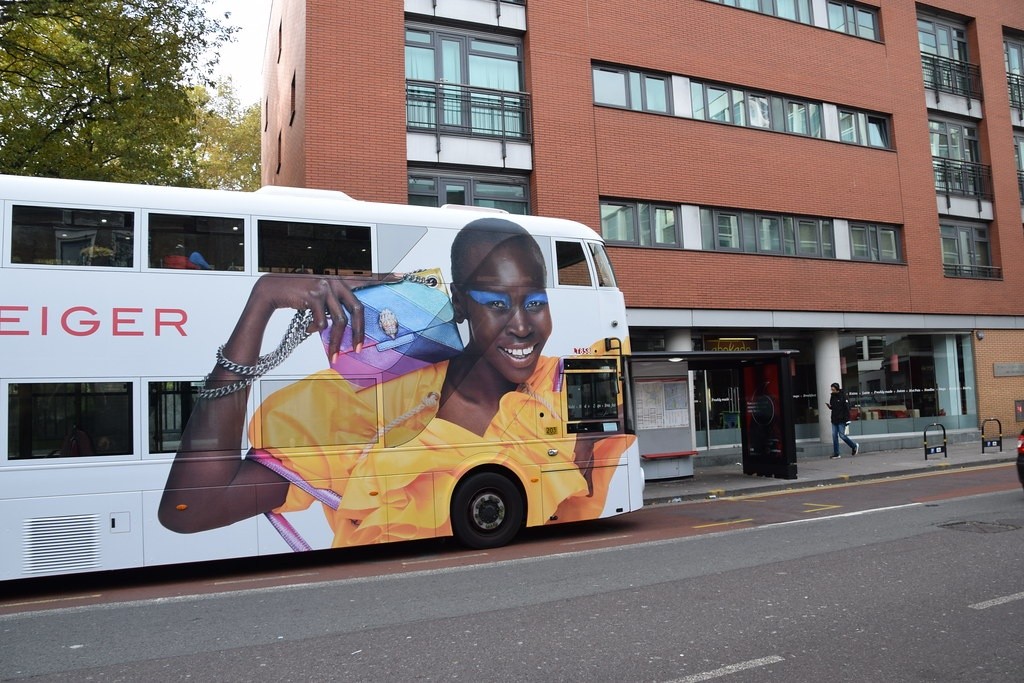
827, 383, 859, 459
158, 218, 636, 547
60, 418, 97, 455
166, 244, 210, 269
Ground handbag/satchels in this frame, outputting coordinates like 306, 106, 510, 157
316, 268, 464, 394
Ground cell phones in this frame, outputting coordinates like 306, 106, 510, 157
315, 268, 455, 359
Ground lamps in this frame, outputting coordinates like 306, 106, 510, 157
791, 358, 796, 376
840, 334, 847, 375
889, 334, 900, 372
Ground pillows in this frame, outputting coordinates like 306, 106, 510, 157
892, 411, 908, 418
850, 409, 858, 421
869, 409, 883, 419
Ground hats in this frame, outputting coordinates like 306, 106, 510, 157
831, 383, 840, 390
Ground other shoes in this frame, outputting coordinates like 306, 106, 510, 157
830, 455, 841, 459
852, 443, 859, 455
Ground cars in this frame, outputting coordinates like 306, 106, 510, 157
1016, 428, 1024, 489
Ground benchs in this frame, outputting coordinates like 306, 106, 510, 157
640, 450, 698, 460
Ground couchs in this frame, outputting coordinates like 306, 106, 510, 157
865, 404, 921, 420
851, 407, 871, 420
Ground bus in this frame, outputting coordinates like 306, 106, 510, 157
1, 173, 646, 586
